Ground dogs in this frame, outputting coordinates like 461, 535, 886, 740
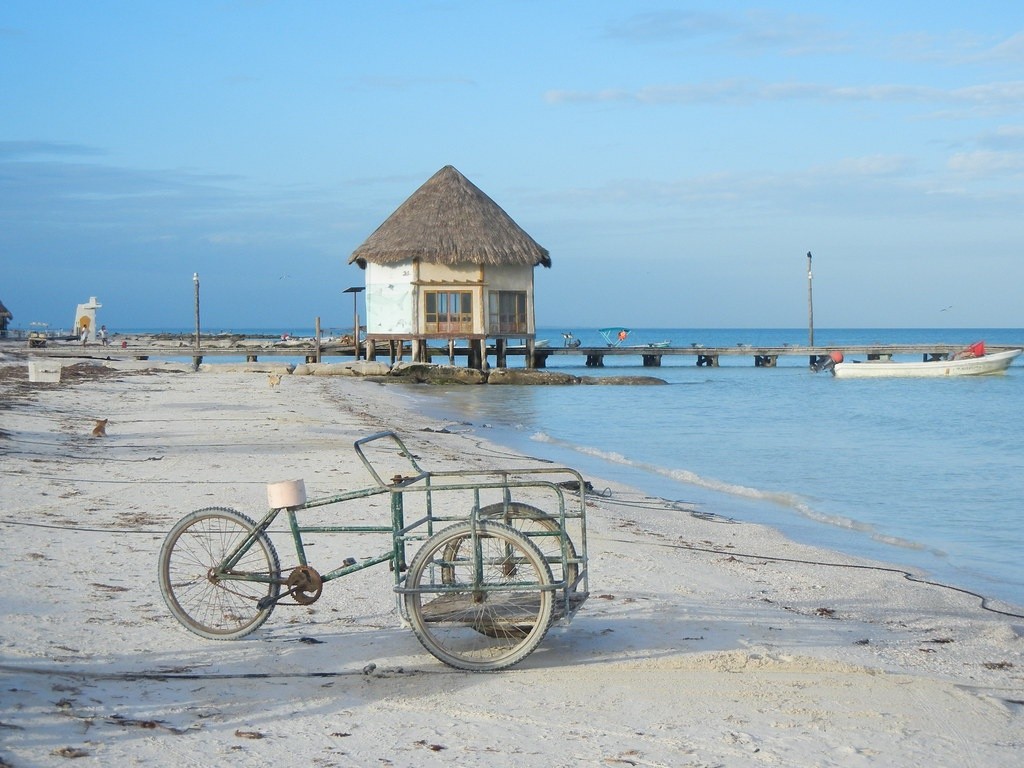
268, 374, 282, 387
93, 419, 108, 437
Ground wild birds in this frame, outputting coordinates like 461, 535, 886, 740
561, 332, 573, 346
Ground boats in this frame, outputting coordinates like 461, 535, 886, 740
486, 339, 550, 349
834, 347, 1022, 377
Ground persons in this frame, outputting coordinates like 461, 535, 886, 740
81, 324, 108, 347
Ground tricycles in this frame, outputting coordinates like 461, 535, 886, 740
156, 429, 590, 677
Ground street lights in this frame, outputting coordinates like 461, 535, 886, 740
807, 250, 814, 347
192, 271, 202, 349
